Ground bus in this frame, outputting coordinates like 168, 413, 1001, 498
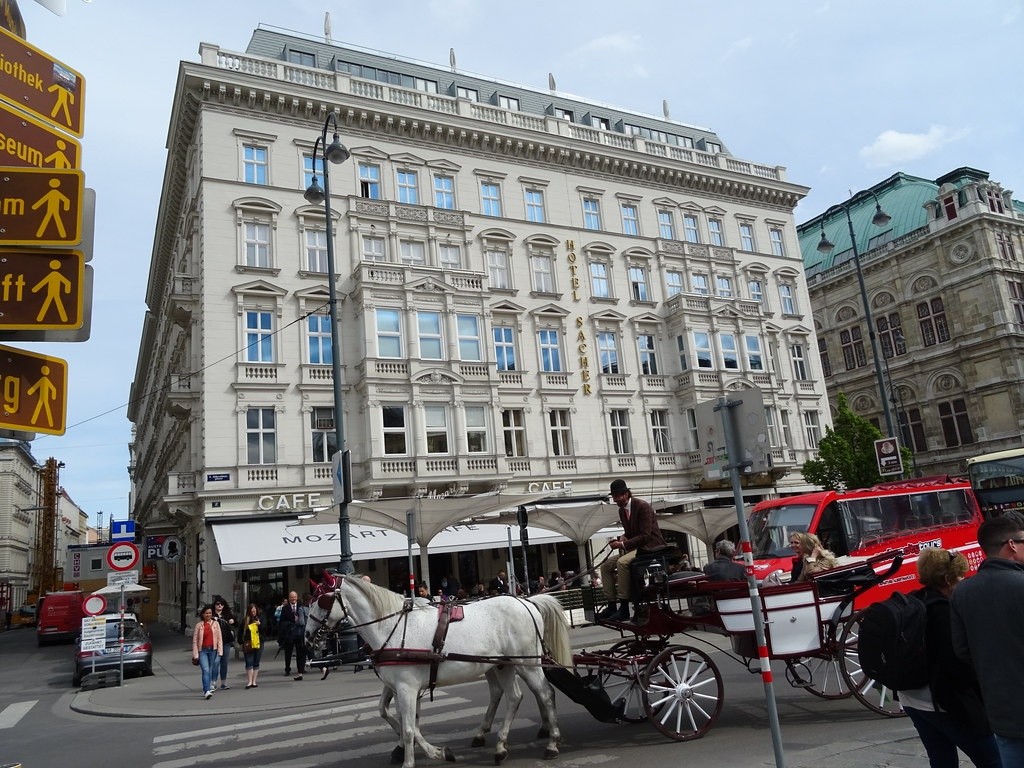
960, 447, 1024, 528
732, 473, 985, 614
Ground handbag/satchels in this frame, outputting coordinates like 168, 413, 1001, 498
248, 622, 260, 649
192, 658, 199, 666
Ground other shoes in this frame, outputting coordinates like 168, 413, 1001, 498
245, 684, 252, 689
598, 608, 617, 618
220, 685, 230, 689
321, 669, 329, 680
607, 606, 630, 621
252, 683, 257, 687
204, 691, 213, 700
210, 684, 218, 692
284, 673, 290, 676
293, 676, 302, 680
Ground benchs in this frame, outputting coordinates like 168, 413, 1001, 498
610, 545, 676, 623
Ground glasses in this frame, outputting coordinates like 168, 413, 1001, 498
216, 603, 223, 606
948, 551, 955, 568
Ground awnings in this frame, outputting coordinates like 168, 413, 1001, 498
211, 499, 624, 572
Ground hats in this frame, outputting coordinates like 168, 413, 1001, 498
608, 479, 631, 495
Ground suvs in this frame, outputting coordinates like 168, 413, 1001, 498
76, 612, 154, 683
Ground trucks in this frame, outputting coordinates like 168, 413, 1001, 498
35, 591, 85, 645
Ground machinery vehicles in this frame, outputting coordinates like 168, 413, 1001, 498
11, 455, 64, 625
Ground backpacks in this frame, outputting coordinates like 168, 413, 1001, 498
217, 619, 235, 644
858, 592, 950, 691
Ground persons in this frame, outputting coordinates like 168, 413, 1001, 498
599, 480, 667, 621
515, 571, 601, 596
399, 570, 509, 600
789, 532, 834, 582
895, 548, 999, 768
362, 576, 371, 582
950, 517, 1024, 768
680, 554, 690, 570
703, 540, 747, 580
275, 591, 330, 680
237, 604, 264, 689
192, 598, 236, 700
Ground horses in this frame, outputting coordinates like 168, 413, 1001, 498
303, 568, 573, 768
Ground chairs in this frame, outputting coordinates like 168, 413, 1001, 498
861, 510, 966, 551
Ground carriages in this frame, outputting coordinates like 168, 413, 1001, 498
303, 476, 911, 767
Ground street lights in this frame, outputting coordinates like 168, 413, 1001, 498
818, 190, 919, 513
304, 110, 360, 576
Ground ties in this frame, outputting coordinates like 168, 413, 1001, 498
625, 509, 629, 521
293, 606, 295, 615
